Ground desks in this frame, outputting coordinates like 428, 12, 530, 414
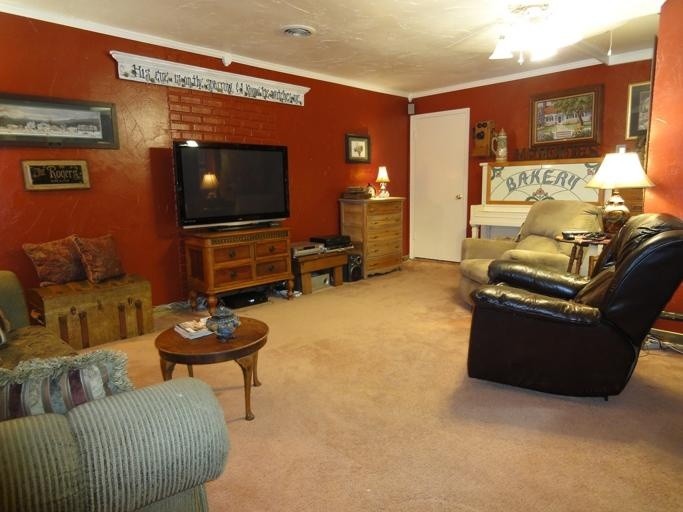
551, 233, 615, 276
292, 248, 349, 295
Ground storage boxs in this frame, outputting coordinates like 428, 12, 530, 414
24, 273, 154, 352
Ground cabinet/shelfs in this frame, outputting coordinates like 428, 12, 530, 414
337, 196, 407, 279
182, 223, 294, 319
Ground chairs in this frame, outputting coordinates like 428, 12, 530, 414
459, 211, 682, 402
459, 195, 604, 313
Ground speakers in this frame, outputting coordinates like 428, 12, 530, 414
344, 242, 362, 282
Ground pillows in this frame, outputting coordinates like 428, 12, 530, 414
70, 233, 124, 283
19, 233, 86, 288
1, 307, 17, 347
0, 348, 134, 422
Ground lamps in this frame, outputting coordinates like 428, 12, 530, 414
485, 3, 558, 68
582, 146, 656, 238
200, 173, 220, 200
374, 166, 390, 199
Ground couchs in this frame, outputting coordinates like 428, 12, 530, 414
0, 270, 229, 512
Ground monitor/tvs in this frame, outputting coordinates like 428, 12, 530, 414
173, 138, 290, 233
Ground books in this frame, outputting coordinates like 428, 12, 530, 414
173, 316, 214, 340
340, 186, 371, 200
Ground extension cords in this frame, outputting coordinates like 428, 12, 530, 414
643, 341, 670, 350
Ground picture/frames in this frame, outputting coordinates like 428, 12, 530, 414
21, 157, 91, 193
624, 80, 651, 141
0, 91, 120, 150
528, 83, 605, 150
343, 133, 371, 164
